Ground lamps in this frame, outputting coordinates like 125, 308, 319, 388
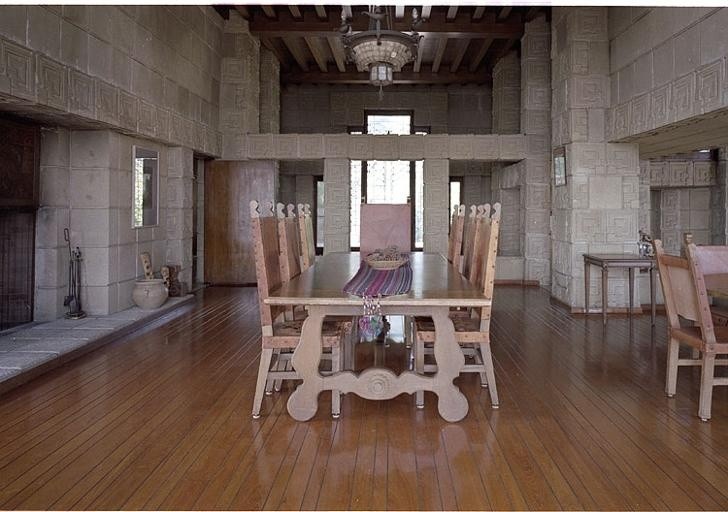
340, 6, 432, 101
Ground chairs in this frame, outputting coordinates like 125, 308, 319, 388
652, 238, 728, 421
402, 202, 499, 413
353, 202, 417, 254
683, 233, 728, 359
249, 201, 364, 418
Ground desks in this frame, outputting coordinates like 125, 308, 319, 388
582, 252, 657, 326
703, 273, 728, 300
584, 326, 664, 389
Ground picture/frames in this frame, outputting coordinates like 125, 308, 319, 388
553, 146, 567, 186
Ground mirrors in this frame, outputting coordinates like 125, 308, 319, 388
132, 145, 160, 230
